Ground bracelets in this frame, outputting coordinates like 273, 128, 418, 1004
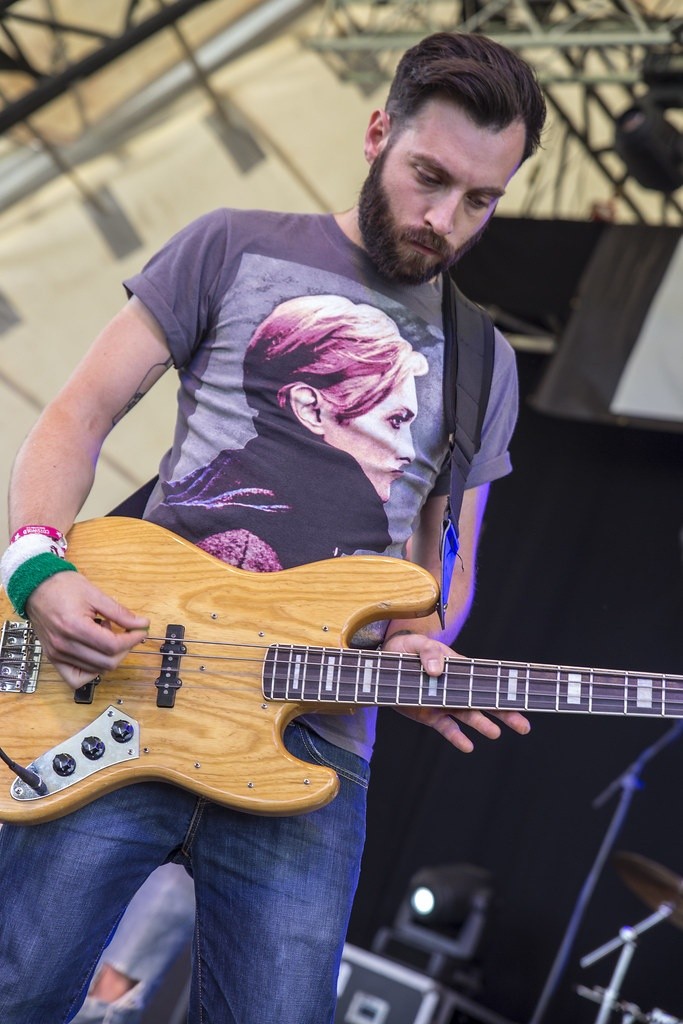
1, 524, 77, 621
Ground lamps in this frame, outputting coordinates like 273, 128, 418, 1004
615, 48, 682, 194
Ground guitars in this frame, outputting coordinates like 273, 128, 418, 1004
0, 515, 683, 827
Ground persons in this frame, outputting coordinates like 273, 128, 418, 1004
0, 30, 548, 1024
69, 862, 196, 1024
